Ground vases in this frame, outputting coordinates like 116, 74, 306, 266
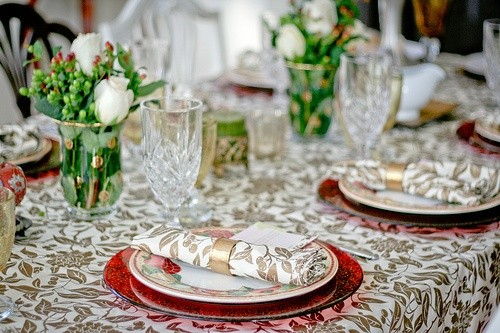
284, 59, 337, 140
54, 119, 127, 221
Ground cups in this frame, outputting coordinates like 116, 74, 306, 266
246, 106, 285, 166
381, 65, 406, 133
119, 35, 171, 158
481, 17, 500, 114
396, 64, 447, 126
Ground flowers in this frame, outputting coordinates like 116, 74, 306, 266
19, 30, 171, 210
267, 0, 371, 133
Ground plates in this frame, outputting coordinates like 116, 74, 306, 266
457, 50, 484, 79
101, 239, 364, 322
455, 119, 500, 156
10, 131, 62, 176
338, 158, 500, 216
317, 173, 500, 228
129, 227, 340, 305
225, 68, 275, 91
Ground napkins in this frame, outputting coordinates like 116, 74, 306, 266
129, 223, 328, 287
332, 162, 499, 208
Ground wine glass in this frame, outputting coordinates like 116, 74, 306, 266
338, 44, 394, 156
132, 97, 204, 238
149, 117, 221, 225
0, 184, 17, 324
412, 0, 447, 63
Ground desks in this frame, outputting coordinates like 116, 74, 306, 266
0, 51, 500, 333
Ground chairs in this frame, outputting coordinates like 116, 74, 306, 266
0, 0, 228, 119
